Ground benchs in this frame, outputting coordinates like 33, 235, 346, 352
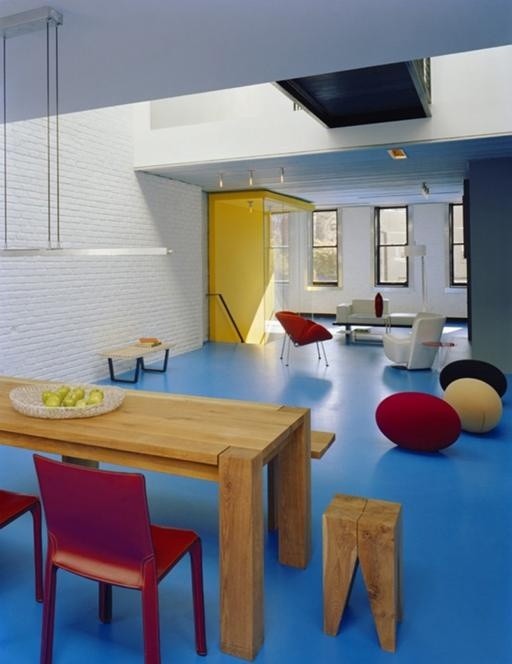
98, 335, 178, 384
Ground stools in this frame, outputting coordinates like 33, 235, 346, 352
319, 494, 410, 652
311, 430, 336, 458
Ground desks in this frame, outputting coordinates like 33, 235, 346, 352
0, 376, 311, 660
333, 320, 413, 346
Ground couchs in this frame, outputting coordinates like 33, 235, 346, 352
335, 297, 390, 321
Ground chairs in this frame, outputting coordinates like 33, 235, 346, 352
383, 311, 447, 370
34, 454, 205, 660
275, 311, 332, 366
1, 490, 45, 603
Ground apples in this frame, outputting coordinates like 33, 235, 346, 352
41, 385, 104, 406
